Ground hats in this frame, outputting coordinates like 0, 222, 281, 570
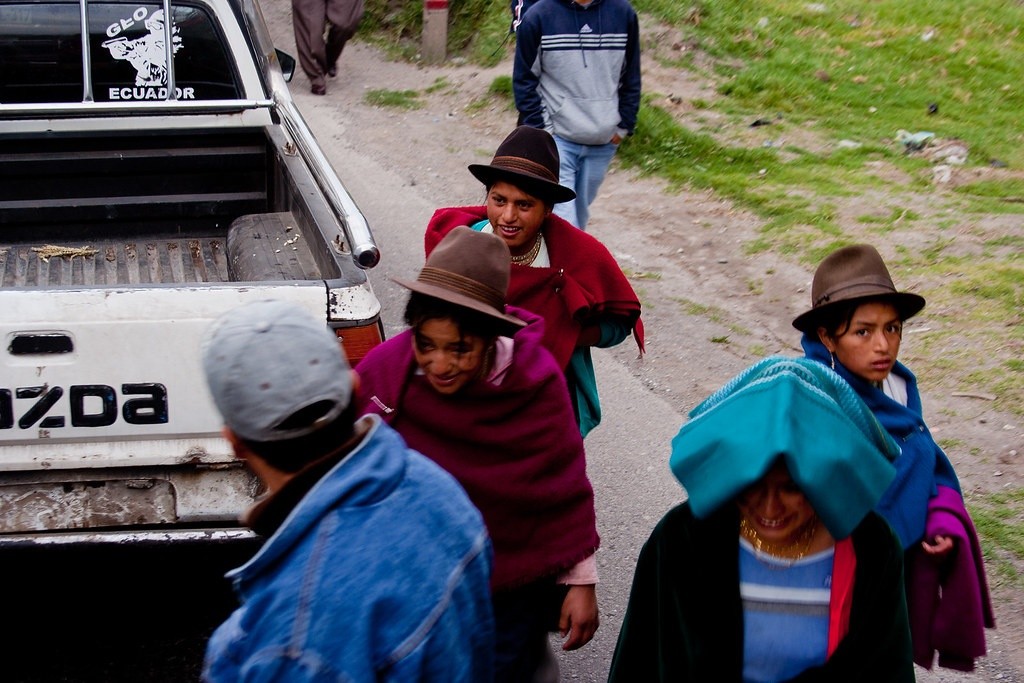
388, 226, 527, 337
468, 125, 576, 203
792, 244, 926, 333
202, 295, 352, 441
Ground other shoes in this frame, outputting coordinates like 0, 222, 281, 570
311, 84, 327, 95
327, 68, 337, 76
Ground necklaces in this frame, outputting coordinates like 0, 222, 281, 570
741, 517, 815, 568
511, 233, 543, 266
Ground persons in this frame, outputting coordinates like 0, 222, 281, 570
606, 357, 917, 683
292, 0, 362, 94
191, 297, 493, 681
793, 245, 998, 673
420, 125, 646, 442
512, 0, 642, 229
349, 226, 603, 682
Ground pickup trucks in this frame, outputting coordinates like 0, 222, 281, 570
0, 0, 385, 542
